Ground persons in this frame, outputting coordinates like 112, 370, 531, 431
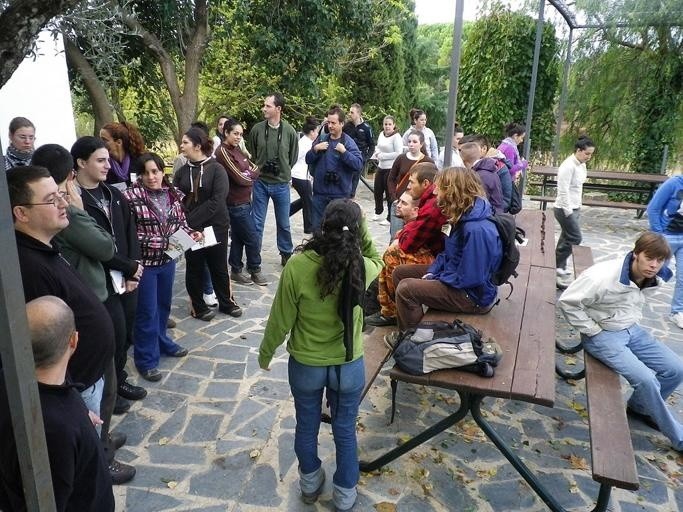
0, 116, 203, 510
558, 232, 682, 462
172, 94, 299, 321
367, 109, 530, 351
255, 197, 386, 512
552, 134, 596, 277
646, 174, 683, 329
289, 103, 376, 237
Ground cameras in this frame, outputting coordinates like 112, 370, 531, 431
262, 157, 280, 176
323, 171, 341, 185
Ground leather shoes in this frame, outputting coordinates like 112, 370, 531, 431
106, 381, 146, 484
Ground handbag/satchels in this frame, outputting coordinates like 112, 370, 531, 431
394, 319, 483, 375
508, 180, 522, 214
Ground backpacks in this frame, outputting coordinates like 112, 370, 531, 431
487, 209, 524, 285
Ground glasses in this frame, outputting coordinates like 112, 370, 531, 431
23, 191, 68, 207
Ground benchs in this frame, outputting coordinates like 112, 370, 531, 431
528, 181, 660, 203
315, 323, 404, 424
530, 195, 647, 223
557, 244, 639, 512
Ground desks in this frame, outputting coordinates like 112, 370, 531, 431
530, 165, 670, 218
357, 210, 556, 510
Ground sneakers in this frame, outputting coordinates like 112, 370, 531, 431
137, 366, 161, 381
379, 219, 390, 225
669, 312, 683, 328
191, 272, 268, 321
365, 314, 397, 326
172, 347, 187, 357
627, 407, 660, 429
302, 468, 325, 504
282, 255, 291, 266
372, 213, 380, 221
557, 266, 571, 276
